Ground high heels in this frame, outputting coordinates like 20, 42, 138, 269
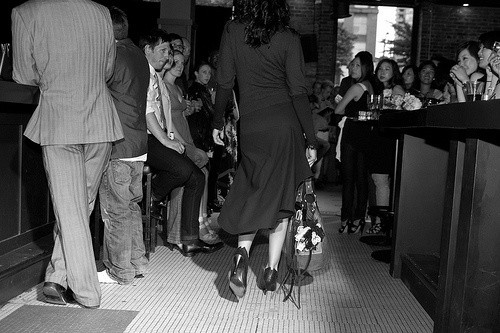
227, 247, 247, 299
263, 268, 278, 293
338, 218, 366, 236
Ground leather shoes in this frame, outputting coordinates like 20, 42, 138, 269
41, 283, 102, 309
181, 241, 224, 257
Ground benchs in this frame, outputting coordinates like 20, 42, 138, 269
95, 168, 167, 260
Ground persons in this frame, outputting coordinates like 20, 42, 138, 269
138, 28, 500, 257
96, 5, 149, 286
11, 0, 125, 309
211, 0, 320, 298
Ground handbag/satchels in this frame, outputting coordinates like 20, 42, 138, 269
286, 177, 328, 273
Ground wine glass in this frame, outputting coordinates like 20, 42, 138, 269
184, 93, 198, 100
206, 144, 215, 153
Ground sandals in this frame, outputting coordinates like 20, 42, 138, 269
198, 213, 219, 241
368, 223, 384, 234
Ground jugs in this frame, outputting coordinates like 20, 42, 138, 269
0, 42, 13, 81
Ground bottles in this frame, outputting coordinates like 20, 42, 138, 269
169, 130, 175, 143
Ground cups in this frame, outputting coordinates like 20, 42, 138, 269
462, 81, 484, 102
490, 41, 500, 65
366, 94, 381, 109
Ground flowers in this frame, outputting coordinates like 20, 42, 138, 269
391, 90, 444, 111
